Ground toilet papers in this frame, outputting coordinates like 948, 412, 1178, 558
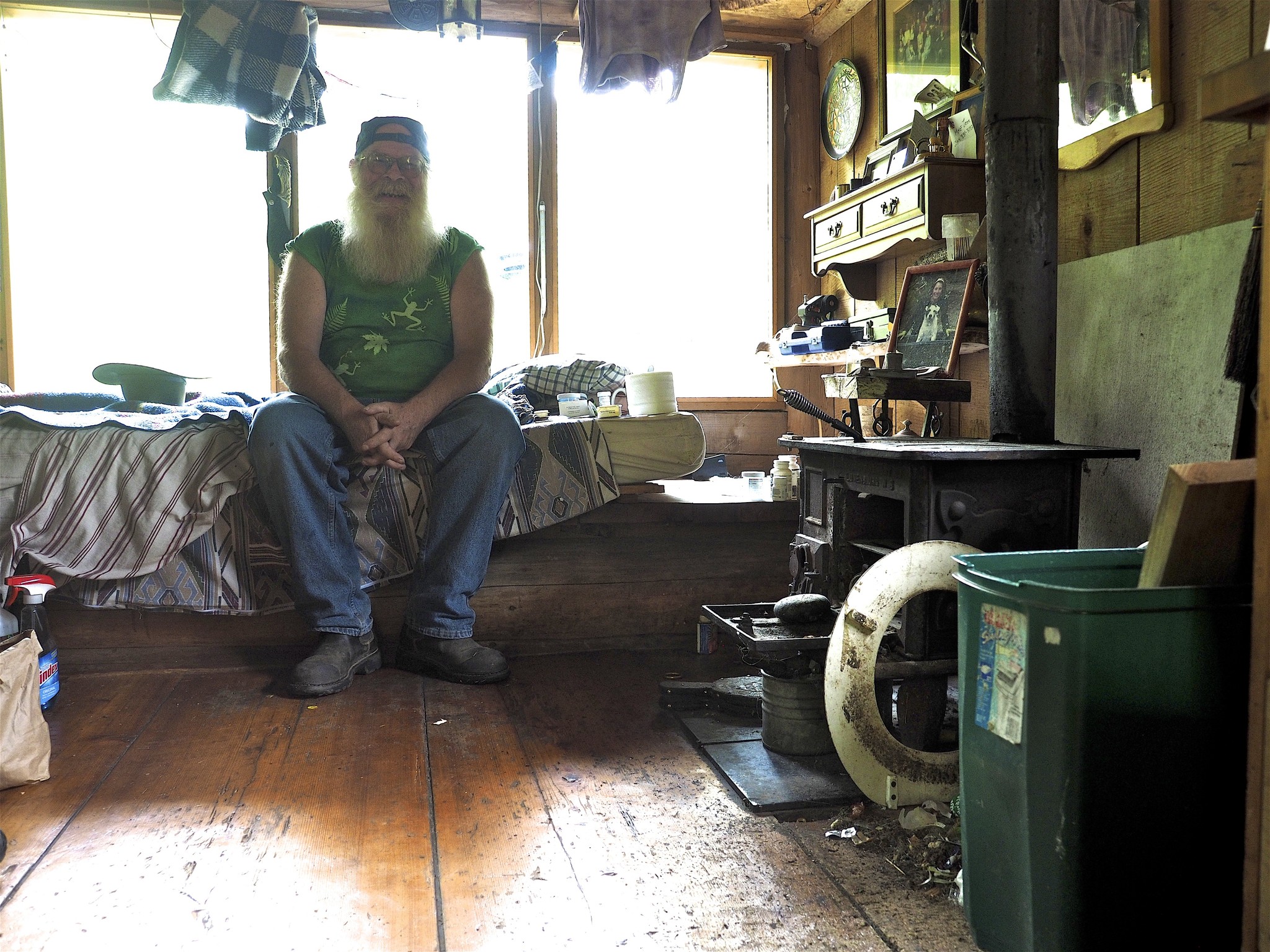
623, 371, 676, 416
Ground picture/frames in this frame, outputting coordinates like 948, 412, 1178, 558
881, 258, 980, 379
862, 0, 986, 184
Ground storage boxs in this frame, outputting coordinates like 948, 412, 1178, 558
779, 308, 896, 356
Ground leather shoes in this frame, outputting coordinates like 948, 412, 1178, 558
289, 625, 382, 697
403, 627, 507, 683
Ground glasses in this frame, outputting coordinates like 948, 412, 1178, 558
357, 152, 427, 179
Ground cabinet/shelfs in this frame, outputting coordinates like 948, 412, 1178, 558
803, 156, 985, 301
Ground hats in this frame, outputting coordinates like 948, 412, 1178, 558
92, 363, 211, 406
356, 117, 429, 166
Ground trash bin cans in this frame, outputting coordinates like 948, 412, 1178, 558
951, 553, 1252, 952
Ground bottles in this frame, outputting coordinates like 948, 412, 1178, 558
742, 471, 766, 501
778, 455, 801, 496
770, 460, 792, 501
597, 391, 611, 406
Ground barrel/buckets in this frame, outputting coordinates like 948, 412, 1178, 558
760, 669, 836, 756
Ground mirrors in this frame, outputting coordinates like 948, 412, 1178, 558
1059, 0, 1174, 171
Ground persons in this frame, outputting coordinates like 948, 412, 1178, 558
907, 276, 948, 342
248, 118, 526, 697
968, 104, 978, 130
897, 0, 951, 64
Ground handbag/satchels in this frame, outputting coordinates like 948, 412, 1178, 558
1, 629, 51, 791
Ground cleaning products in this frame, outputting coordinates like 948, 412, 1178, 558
3, 574, 62, 713
